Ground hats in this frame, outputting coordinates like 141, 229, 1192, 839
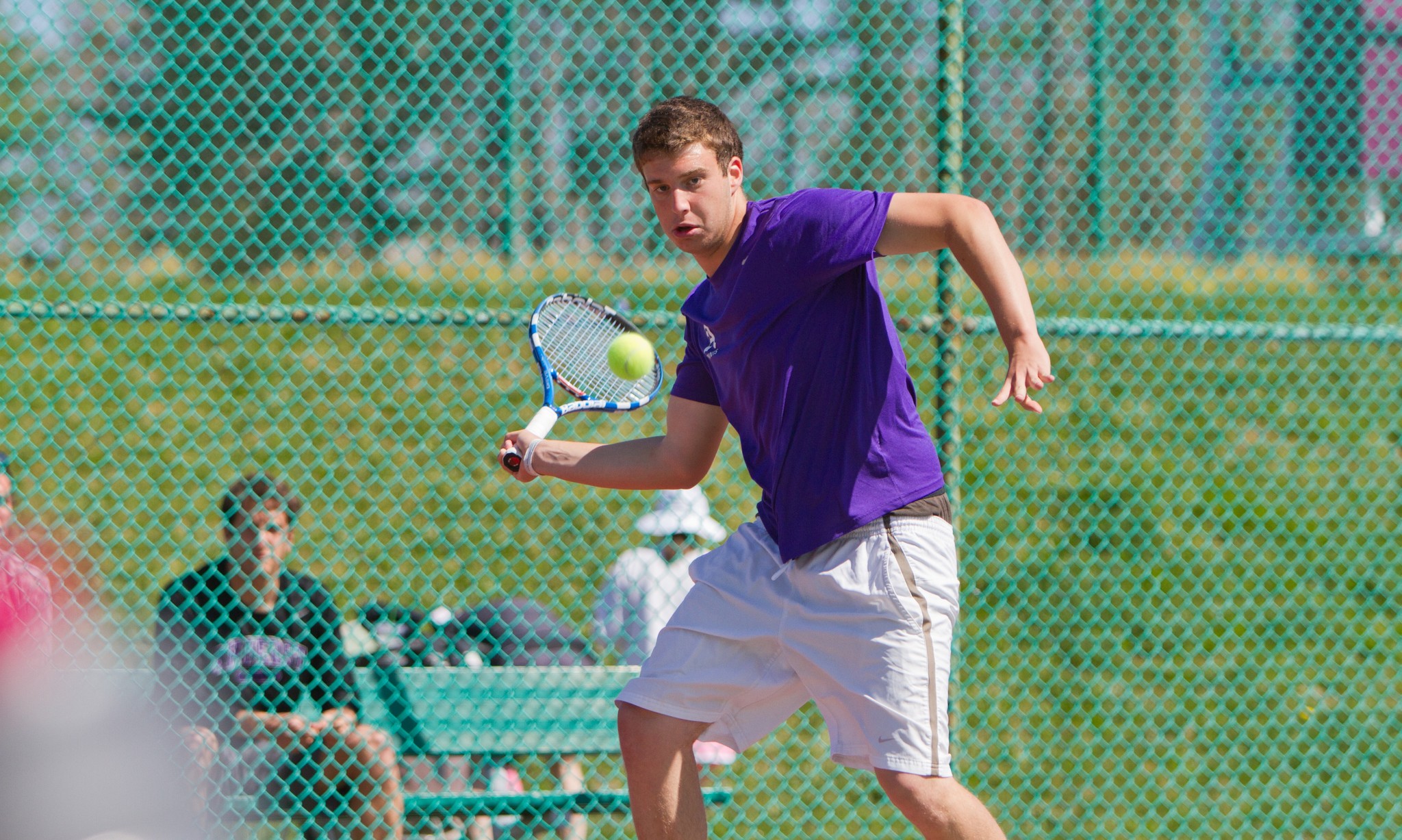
634, 485, 728, 543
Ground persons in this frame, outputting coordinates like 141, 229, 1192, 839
400, 596, 594, 839
144, 471, 411, 839
488, 86, 1057, 839
1, 443, 57, 695
592, 484, 731, 665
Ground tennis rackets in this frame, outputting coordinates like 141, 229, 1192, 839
502, 293, 665, 473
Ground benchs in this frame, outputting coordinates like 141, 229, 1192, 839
111, 663, 734, 820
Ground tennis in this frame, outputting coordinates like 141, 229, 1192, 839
607, 332, 656, 382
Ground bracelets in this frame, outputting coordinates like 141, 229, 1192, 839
521, 437, 548, 477
